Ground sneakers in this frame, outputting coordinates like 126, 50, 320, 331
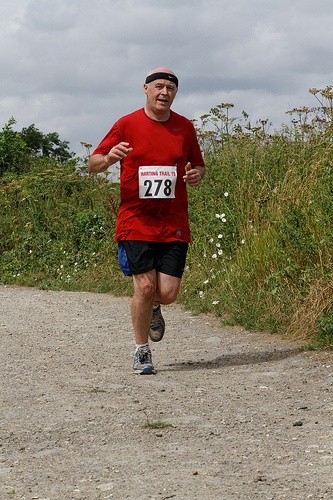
148, 305, 165, 342
132, 345, 156, 374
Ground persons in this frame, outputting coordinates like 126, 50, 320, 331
87, 68, 206, 375
